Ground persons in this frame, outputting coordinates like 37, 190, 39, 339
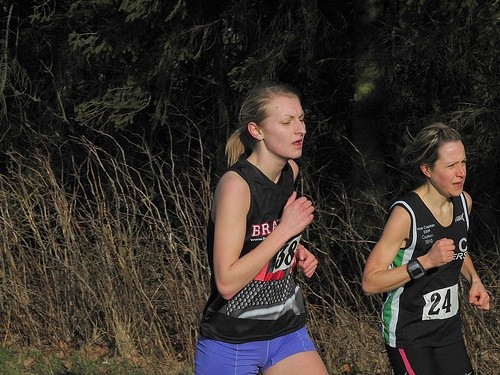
360, 121, 491, 375
189, 84, 335, 375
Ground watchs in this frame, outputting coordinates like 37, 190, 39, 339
406, 257, 430, 279
